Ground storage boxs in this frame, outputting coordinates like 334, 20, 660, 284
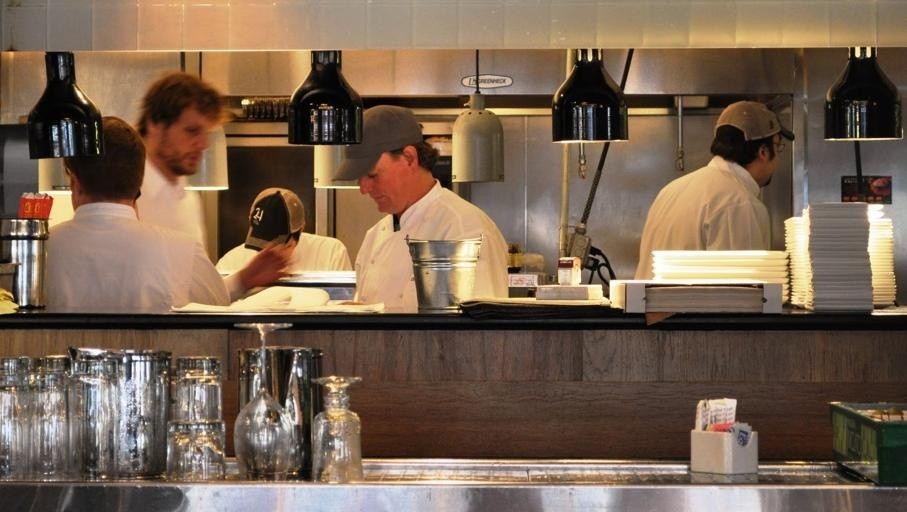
689, 427, 762, 477
825, 401, 907, 487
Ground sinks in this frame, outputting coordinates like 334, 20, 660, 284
2, 482, 186, 510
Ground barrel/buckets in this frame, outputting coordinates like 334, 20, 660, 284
406, 234, 484, 315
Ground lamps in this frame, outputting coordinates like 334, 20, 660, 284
448, 48, 507, 184
25, 50, 105, 160
285, 49, 366, 146
550, 48, 635, 147
179, 50, 236, 192
310, 144, 366, 196
822, 46, 905, 144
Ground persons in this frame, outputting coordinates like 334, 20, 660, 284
332, 103, 511, 314
43, 115, 232, 314
632, 97, 796, 283
211, 184, 353, 280
131, 72, 296, 303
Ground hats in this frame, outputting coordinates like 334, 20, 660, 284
330, 105, 423, 183
244, 186, 305, 252
713, 100, 795, 142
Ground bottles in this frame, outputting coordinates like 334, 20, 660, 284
0, 352, 225, 484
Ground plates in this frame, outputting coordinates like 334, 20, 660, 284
650, 201, 896, 314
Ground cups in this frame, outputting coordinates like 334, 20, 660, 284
0, 217, 48, 310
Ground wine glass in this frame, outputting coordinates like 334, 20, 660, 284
229, 317, 366, 486
